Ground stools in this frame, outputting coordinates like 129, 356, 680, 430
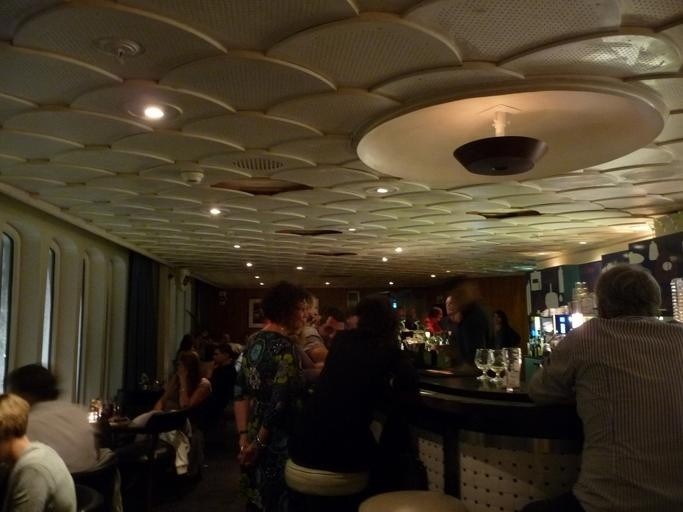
282, 453, 372, 500
358, 489, 466, 511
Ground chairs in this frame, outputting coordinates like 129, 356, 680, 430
143, 406, 190, 448
77, 482, 102, 511
70, 459, 114, 501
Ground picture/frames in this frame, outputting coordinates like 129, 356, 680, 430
246, 296, 268, 329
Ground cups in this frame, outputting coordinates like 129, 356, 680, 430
503, 348, 521, 392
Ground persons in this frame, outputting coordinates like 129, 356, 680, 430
154, 350, 212, 410
172, 327, 213, 360
526, 263, 683, 512
0, 392, 76, 512
4, 363, 98, 475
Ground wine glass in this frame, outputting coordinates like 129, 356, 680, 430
475, 348, 495, 380
487, 350, 508, 381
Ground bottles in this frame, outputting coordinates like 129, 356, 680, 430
571, 281, 590, 313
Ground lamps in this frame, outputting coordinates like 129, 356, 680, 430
122, 96, 184, 125
